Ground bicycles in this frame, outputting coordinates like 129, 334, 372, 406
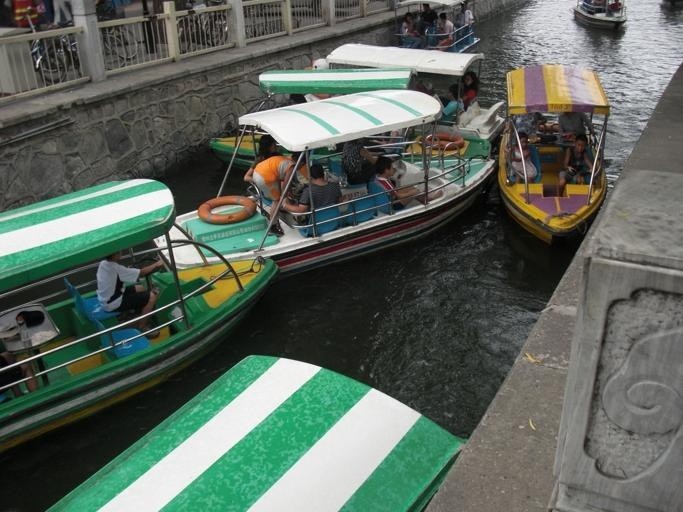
27, 0, 267, 85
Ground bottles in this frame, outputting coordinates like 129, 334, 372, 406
16, 315, 32, 341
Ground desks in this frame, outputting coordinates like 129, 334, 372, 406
0, 303, 60, 387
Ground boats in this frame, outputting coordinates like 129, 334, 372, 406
392, 0, 480, 59
570, 0, 627, 30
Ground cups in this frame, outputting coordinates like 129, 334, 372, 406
323, 168, 330, 179
337, 173, 347, 187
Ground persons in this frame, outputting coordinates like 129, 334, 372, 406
0, 351, 39, 398
610, 0, 622, 11
96, 250, 164, 338
245, 133, 443, 225
400, 2, 474, 52
511, 112, 596, 188
0, 0, 128, 48
416, 72, 477, 126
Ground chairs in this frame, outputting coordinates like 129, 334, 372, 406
327, 158, 367, 189
448, 32, 474, 52
368, 181, 411, 215
64, 276, 121, 322
568, 147, 593, 184
341, 193, 376, 224
297, 204, 341, 238
470, 102, 504, 128
93, 318, 153, 360
399, 28, 414, 47
508, 144, 542, 183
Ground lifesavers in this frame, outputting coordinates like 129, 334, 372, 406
198, 195, 256, 224
425, 133, 464, 151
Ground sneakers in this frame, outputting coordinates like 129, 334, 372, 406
266, 224, 284, 237
139, 326, 161, 339
